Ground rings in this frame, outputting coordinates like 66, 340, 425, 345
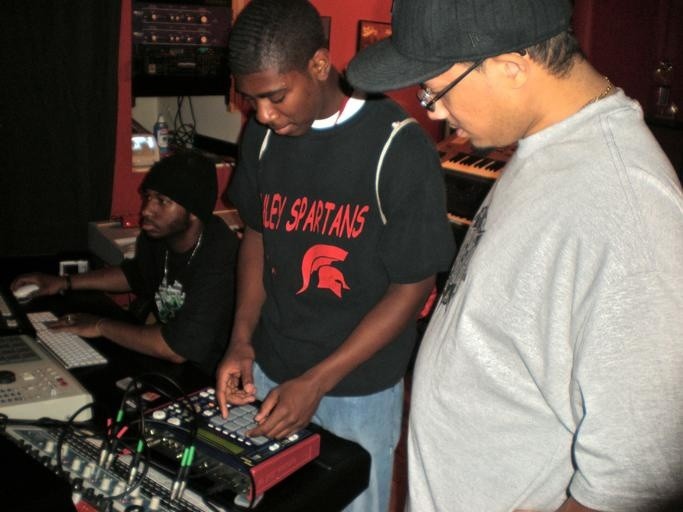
64, 314, 72, 321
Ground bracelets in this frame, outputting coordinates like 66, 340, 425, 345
64, 274, 72, 291
95, 316, 111, 335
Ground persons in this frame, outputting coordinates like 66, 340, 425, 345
214, 1, 455, 511
345, 1, 683, 510
10, 153, 241, 368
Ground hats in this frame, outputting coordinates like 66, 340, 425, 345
139, 151, 218, 215
344, 1, 572, 93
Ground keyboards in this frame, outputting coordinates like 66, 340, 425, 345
27, 312, 108, 370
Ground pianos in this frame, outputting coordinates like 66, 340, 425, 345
436, 132, 517, 232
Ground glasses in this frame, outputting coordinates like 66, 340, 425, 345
415, 47, 526, 112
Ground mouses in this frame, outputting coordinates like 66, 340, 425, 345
13, 284, 40, 300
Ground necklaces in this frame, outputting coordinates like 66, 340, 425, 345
163, 231, 204, 294
581, 74, 612, 108
